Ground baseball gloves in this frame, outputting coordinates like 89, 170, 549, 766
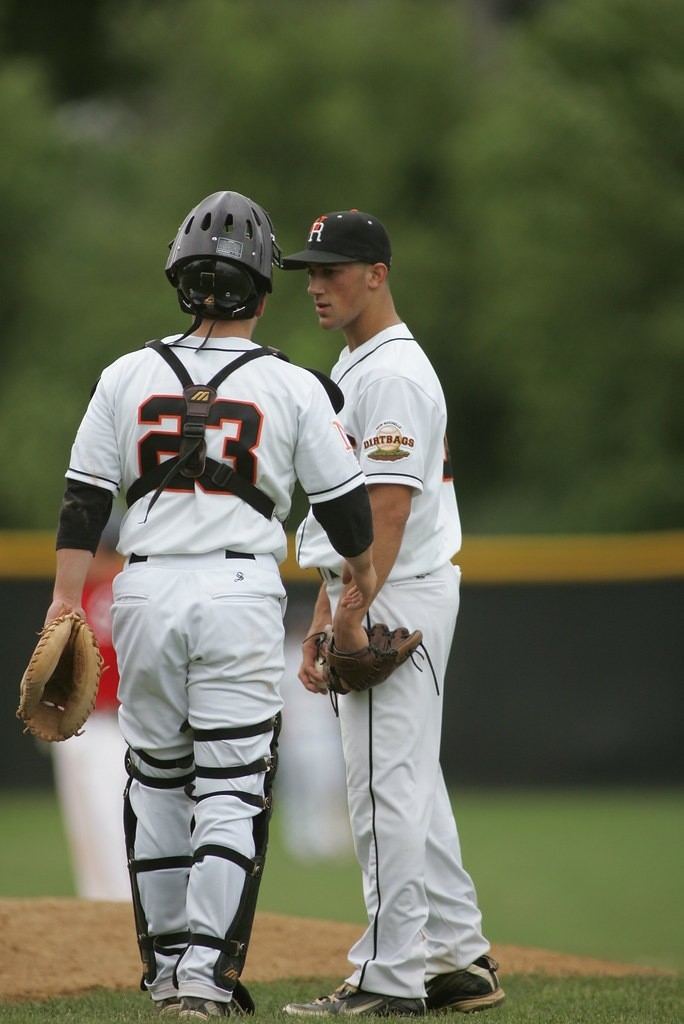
17, 608, 104, 745
316, 622, 423, 696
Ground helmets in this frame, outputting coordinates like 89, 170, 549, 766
165, 191, 282, 294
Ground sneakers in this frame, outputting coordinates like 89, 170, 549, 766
285, 981, 426, 1016
424, 955, 505, 1012
157, 992, 243, 1020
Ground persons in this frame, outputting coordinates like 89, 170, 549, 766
50, 516, 140, 905
41, 183, 378, 1019
272, 208, 512, 1019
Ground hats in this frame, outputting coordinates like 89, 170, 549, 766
282, 209, 392, 270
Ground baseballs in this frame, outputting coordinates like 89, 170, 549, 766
313, 655, 331, 686
